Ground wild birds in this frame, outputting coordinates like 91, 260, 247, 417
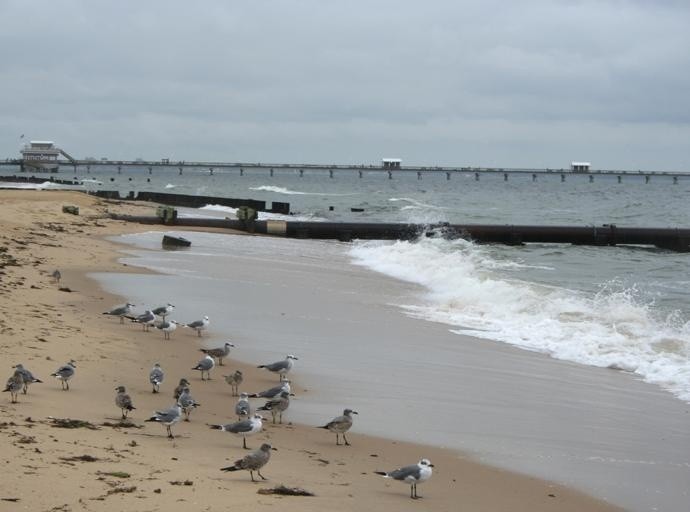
52, 358, 77, 391
144, 402, 183, 438
54, 268, 62, 283
206, 354, 300, 484
198, 342, 236, 366
178, 386, 201, 421
192, 352, 216, 381
173, 378, 189, 399
10, 363, 44, 395
374, 457, 434, 501
113, 385, 136, 419
3, 369, 24, 403
315, 407, 359, 446
146, 363, 164, 393
102, 302, 210, 340
